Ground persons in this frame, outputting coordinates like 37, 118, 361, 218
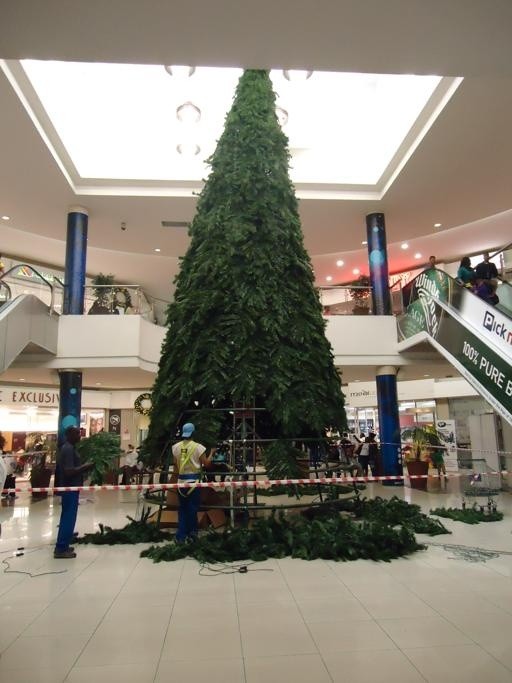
457, 256, 478, 293
172, 421, 219, 543
53, 425, 97, 558
1, 430, 9, 501
473, 252, 501, 298
423, 255, 436, 272
341, 431, 451, 482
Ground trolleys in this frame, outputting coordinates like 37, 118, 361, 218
457, 458, 502, 517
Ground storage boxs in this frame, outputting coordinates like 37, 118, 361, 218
147, 487, 226, 530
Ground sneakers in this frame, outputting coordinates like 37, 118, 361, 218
53, 546, 77, 558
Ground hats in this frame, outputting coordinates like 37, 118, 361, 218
181, 422, 196, 438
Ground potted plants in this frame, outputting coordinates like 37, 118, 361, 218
401, 425, 445, 489
91, 273, 114, 314
18, 433, 56, 498
348, 275, 372, 315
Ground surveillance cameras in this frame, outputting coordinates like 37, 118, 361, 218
121, 223, 127, 231
